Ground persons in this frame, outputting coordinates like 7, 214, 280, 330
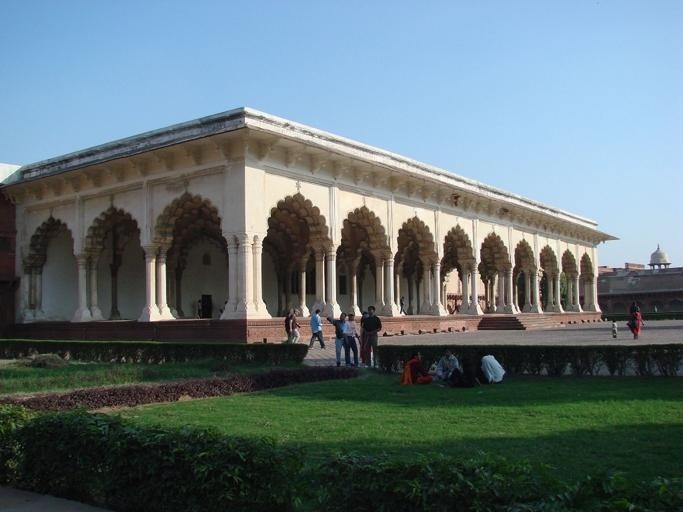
450, 298, 460, 314
197, 299, 202, 319
486, 301, 490, 310
477, 351, 508, 384
326, 312, 354, 368
345, 313, 361, 368
363, 305, 383, 369
403, 350, 433, 385
627, 301, 641, 339
360, 312, 369, 367
307, 309, 328, 351
432, 348, 464, 381
287, 309, 301, 344
400, 295, 408, 315
